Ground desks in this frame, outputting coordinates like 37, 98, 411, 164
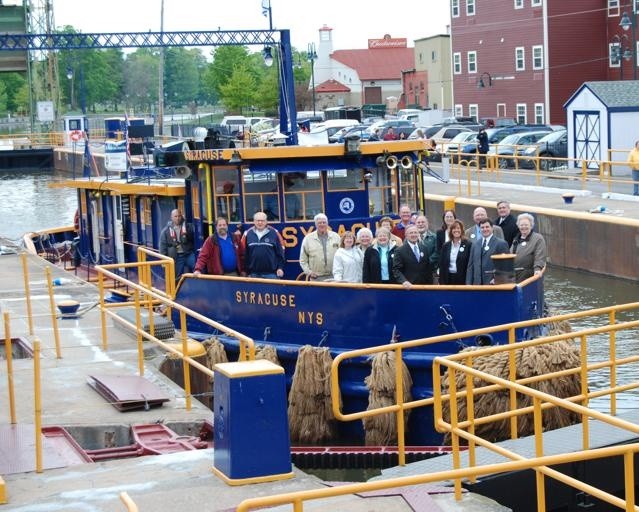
65, 56, 85, 115
610, 34, 632, 80
292, 51, 301, 73
263, 35, 281, 68
618, 1, 637, 81
306, 42, 319, 117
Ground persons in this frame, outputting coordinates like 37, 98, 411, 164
240, 213, 286, 279
629, 140, 639, 196
194, 216, 246, 278
158, 208, 197, 283
338, 129, 347, 143
368, 122, 427, 141
193, 125, 208, 150
476, 128, 490, 166
300, 201, 548, 291
299, 125, 308, 132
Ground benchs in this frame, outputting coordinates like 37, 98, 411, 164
83, 131, 91, 181
122, 112, 132, 166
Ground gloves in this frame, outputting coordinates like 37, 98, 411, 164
23, 110, 584, 468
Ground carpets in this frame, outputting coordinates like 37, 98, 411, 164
481, 238, 487, 252
413, 245, 420, 263
